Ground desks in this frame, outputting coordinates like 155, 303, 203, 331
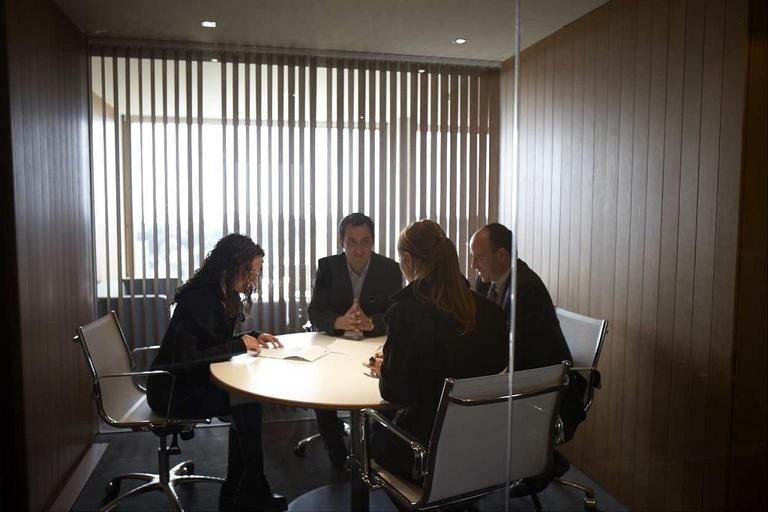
207, 326, 414, 511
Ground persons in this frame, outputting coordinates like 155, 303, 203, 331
147, 233, 287, 511
469, 223, 587, 498
362, 221, 509, 488
307, 214, 404, 466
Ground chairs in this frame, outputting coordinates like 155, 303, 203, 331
97, 277, 186, 386
294, 304, 370, 462
517, 306, 611, 512
346, 355, 574, 511
72, 306, 236, 511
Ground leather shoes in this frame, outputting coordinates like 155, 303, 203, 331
509, 452, 570, 498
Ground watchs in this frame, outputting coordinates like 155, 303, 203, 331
368, 318, 375, 335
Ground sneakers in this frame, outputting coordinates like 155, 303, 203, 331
237, 478, 288, 512
216, 481, 239, 511
